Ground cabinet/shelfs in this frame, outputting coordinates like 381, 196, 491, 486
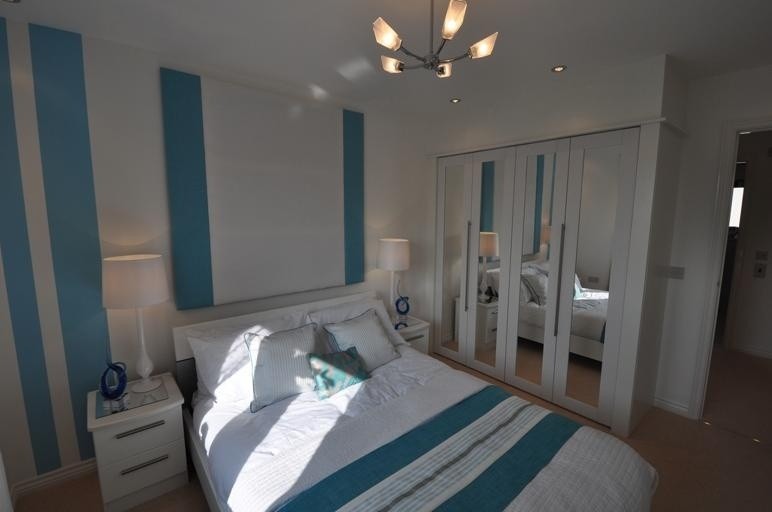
505, 125, 641, 427
433, 147, 515, 387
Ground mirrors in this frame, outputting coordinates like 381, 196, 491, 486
564, 143, 625, 408
471, 160, 504, 369
514, 153, 556, 384
440, 164, 466, 352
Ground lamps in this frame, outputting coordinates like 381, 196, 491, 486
479, 232, 499, 274
100, 254, 170, 394
372, 0, 499, 78
376, 238, 410, 308
543, 225, 551, 243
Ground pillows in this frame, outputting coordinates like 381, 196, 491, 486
520, 264, 582, 303
244, 322, 317, 412
306, 346, 371, 400
323, 307, 401, 372
186, 309, 313, 403
307, 294, 411, 349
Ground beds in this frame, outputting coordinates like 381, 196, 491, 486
477, 255, 610, 362
172, 280, 659, 511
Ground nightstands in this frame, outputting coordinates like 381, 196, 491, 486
388, 315, 431, 354
86, 372, 189, 512
454, 295, 499, 350
